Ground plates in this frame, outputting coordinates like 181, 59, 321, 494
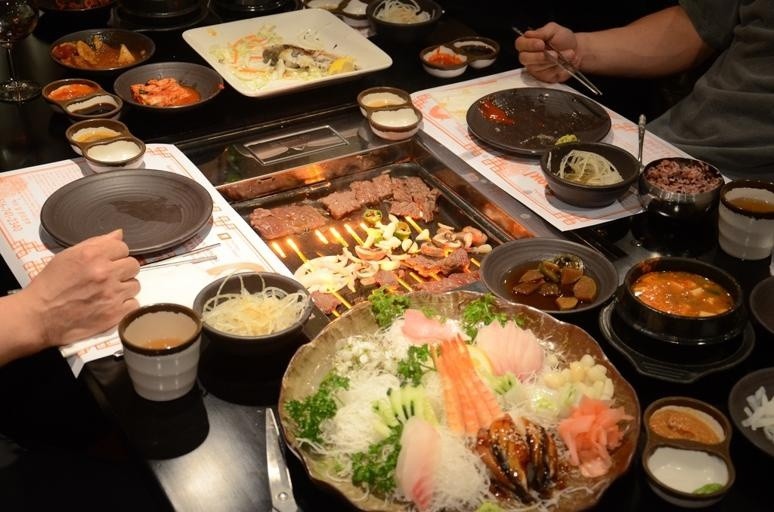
420, 36, 502, 79
43, 76, 147, 173
182, 8, 396, 99
642, 396, 738, 510
597, 299, 758, 387
41, 168, 213, 257
354, 83, 424, 140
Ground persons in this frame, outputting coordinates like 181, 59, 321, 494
0, 228, 141, 369
514, 1, 773, 186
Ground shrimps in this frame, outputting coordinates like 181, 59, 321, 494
431, 332, 502, 433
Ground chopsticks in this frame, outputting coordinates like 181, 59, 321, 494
512, 22, 604, 96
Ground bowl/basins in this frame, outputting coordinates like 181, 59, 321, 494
615, 255, 744, 361
465, 86, 612, 158
368, 0, 446, 37
50, 29, 157, 76
479, 239, 620, 314
727, 366, 774, 458
276, 291, 642, 511
638, 156, 726, 224
114, 61, 224, 113
544, 142, 641, 208
194, 272, 317, 346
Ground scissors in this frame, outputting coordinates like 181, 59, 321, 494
261, 408, 301, 512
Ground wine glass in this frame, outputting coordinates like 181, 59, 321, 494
0, 0, 44, 102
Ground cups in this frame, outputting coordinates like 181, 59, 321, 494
119, 300, 202, 401
719, 176, 774, 264
